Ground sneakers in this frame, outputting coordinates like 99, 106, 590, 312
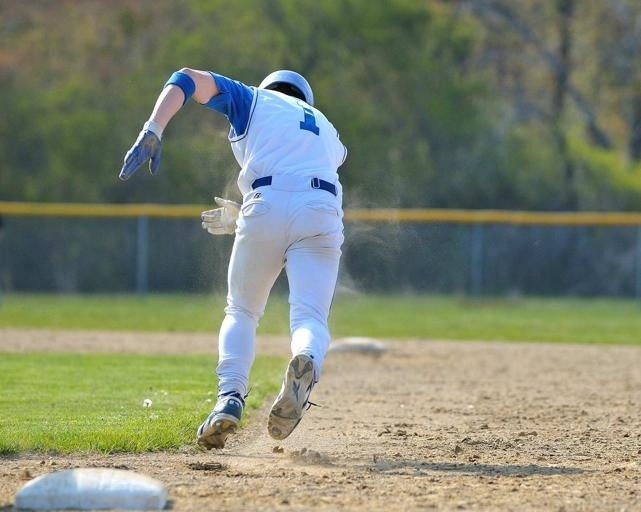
266, 351, 317, 442
195, 392, 245, 451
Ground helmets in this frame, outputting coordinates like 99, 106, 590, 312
258, 68, 315, 106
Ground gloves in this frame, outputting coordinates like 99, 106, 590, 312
200, 195, 243, 236
118, 127, 161, 182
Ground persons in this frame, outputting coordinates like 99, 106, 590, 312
118, 65, 350, 451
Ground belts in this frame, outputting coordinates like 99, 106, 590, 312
252, 173, 338, 197
